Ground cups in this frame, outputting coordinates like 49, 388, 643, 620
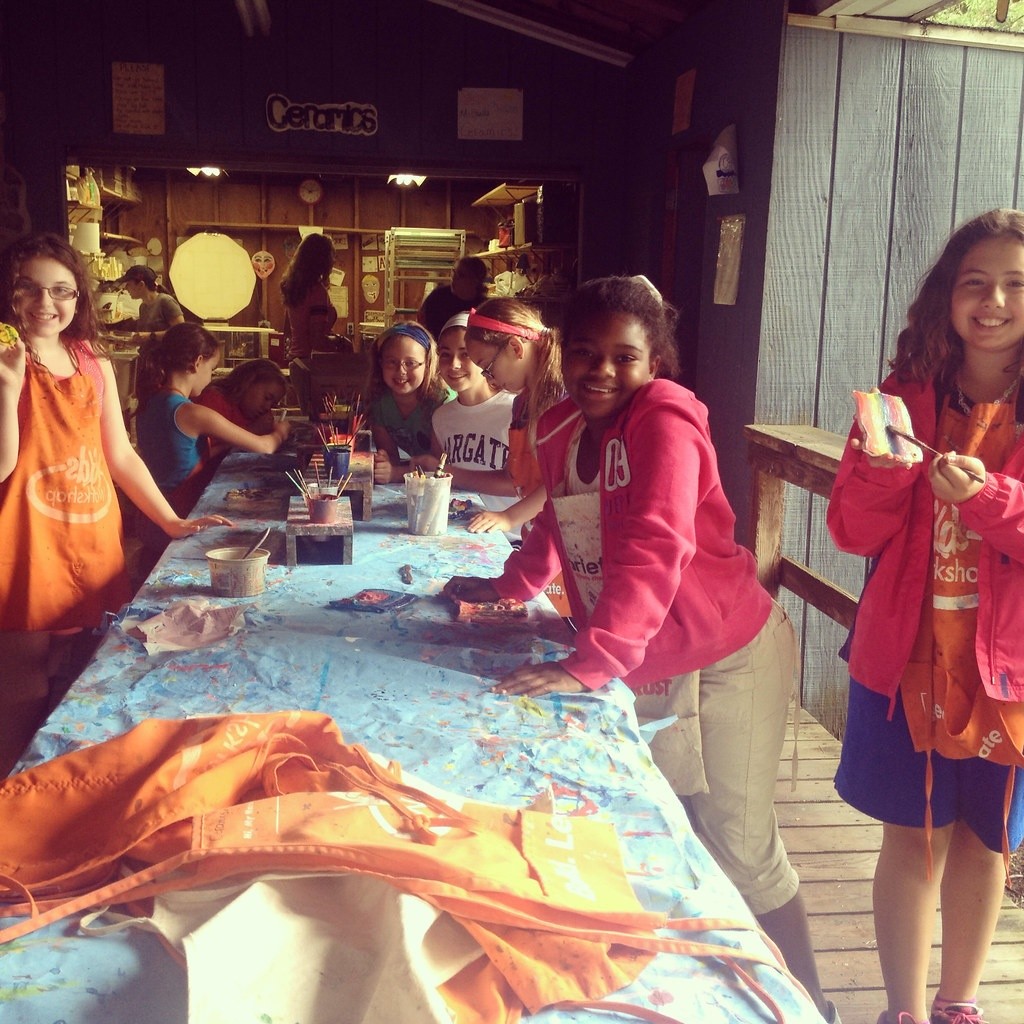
321, 443, 351, 456
331, 404, 352, 412
329, 435, 355, 455
403, 471, 453, 536
301, 482, 340, 524
323, 450, 350, 480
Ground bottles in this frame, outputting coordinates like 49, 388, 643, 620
78, 167, 101, 206
79, 252, 124, 279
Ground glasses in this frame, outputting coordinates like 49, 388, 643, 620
480, 336, 527, 380
15, 280, 81, 301
450, 269, 473, 284
381, 357, 427, 373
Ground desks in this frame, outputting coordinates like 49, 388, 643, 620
1, 413, 828, 1024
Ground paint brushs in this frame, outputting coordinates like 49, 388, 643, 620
317, 413, 368, 453
322, 392, 338, 417
242, 526, 270, 559
888, 426, 986, 485
285, 462, 353, 498
347, 390, 361, 417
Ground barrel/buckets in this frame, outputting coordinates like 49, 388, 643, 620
204, 547, 270, 597
95, 292, 119, 321
110, 353, 139, 411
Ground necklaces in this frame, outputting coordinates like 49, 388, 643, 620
166, 386, 186, 398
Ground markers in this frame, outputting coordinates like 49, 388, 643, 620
279, 409, 287, 422
408, 453, 452, 536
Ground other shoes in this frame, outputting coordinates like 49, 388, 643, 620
877, 1010, 930, 1024
931, 1004, 989, 1024
827, 1000, 843, 1024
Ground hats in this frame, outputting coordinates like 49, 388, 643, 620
115, 264, 158, 284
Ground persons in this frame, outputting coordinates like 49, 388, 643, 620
365, 320, 449, 485
191, 357, 286, 435
443, 274, 835, 1024
431, 311, 526, 512
418, 257, 489, 345
116, 265, 185, 408
135, 323, 291, 591
279, 233, 337, 417
410, 297, 566, 533
0, 237, 230, 727
825, 209, 1024, 1023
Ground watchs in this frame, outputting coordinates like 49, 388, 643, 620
150, 332, 156, 341
